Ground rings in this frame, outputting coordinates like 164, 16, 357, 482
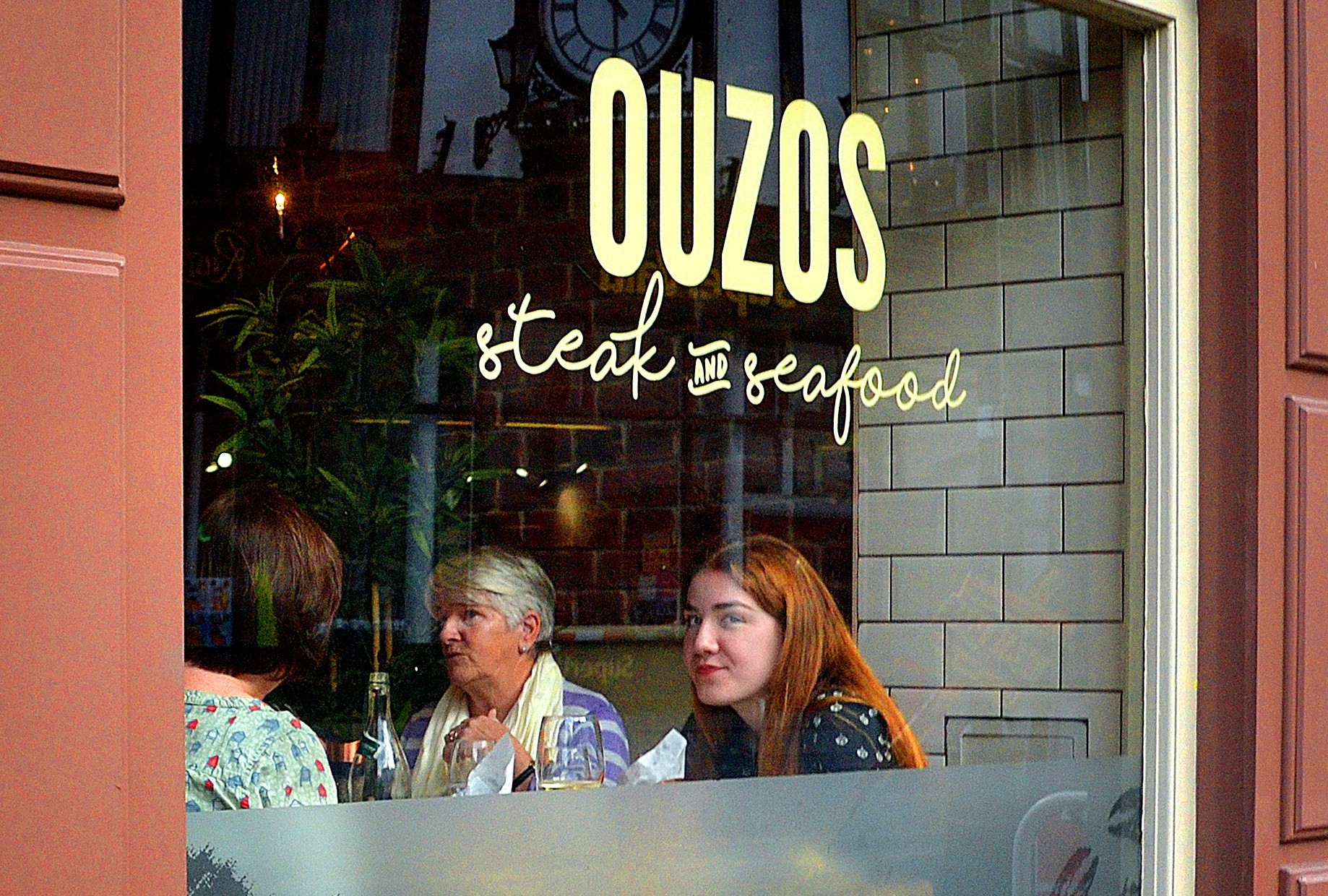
450, 730, 457, 741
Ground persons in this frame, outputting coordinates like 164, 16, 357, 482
391, 543, 626, 794
183, 483, 343, 812
653, 530, 926, 776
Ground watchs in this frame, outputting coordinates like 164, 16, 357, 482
511, 760, 537, 793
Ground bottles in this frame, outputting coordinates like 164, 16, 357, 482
348, 672, 411, 802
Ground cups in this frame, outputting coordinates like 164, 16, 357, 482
449, 741, 497, 797
535, 715, 605, 791
